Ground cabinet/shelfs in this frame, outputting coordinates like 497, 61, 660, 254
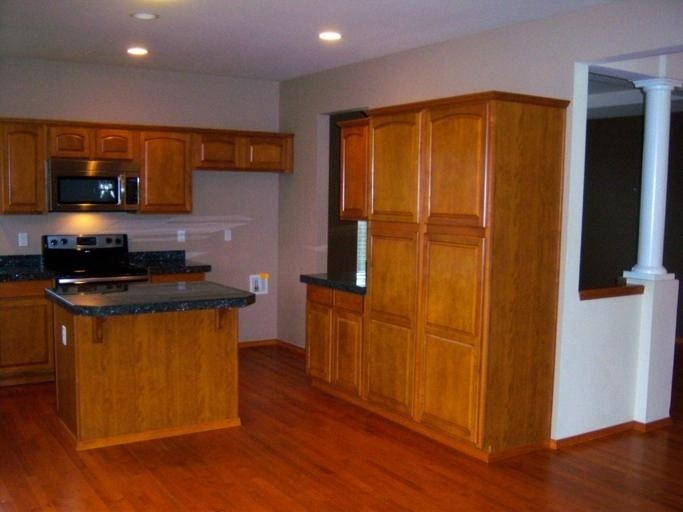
307, 284, 360, 396
337, 116, 368, 221
1, 273, 205, 386
365, 90, 566, 459
0, 118, 294, 216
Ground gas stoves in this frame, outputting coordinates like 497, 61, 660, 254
41, 261, 149, 282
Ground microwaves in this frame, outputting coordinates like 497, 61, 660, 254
45, 158, 140, 212
